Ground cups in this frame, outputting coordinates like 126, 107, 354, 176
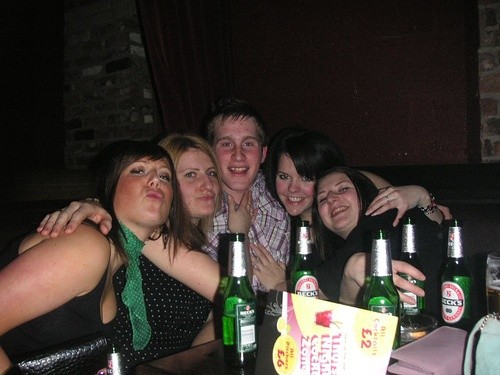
396, 312, 438, 348
485, 251, 500, 321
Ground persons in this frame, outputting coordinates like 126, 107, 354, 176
0, 100, 459, 375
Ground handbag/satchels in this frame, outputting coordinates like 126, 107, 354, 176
388, 324, 469, 375
11, 334, 112, 374
461, 312, 500, 375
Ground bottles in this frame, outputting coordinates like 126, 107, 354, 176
106, 344, 124, 375
289, 221, 319, 298
220, 233, 258, 371
442, 219, 472, 332
397, 216, 424, 315
363, 229, 400, 365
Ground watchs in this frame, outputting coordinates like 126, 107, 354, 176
417, 192, 438, 211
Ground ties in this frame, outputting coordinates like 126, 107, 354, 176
115, 219, 152, 352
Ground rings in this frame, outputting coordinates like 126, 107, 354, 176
385, 195, 389, 202
247, 204, 251, 207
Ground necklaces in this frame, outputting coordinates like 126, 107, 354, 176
230, 189, 245, 211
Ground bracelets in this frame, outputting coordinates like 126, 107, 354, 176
85, 197, 100, 202
425, 206, 437, 215
